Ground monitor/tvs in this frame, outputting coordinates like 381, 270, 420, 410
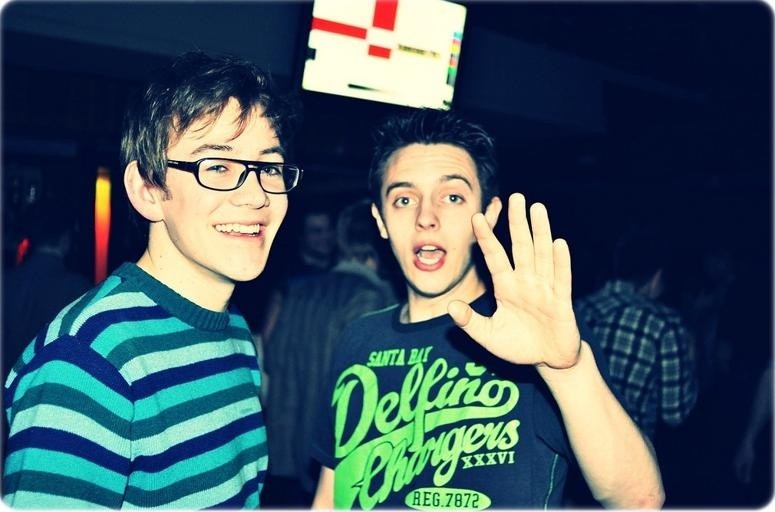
294, 0, 471, 117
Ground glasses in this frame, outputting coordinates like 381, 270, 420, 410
168, 154, 307, 196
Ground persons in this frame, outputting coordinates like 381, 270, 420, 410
1, 54, 308, 508
308, 106, 668, 509
3, 192, 769, 507
